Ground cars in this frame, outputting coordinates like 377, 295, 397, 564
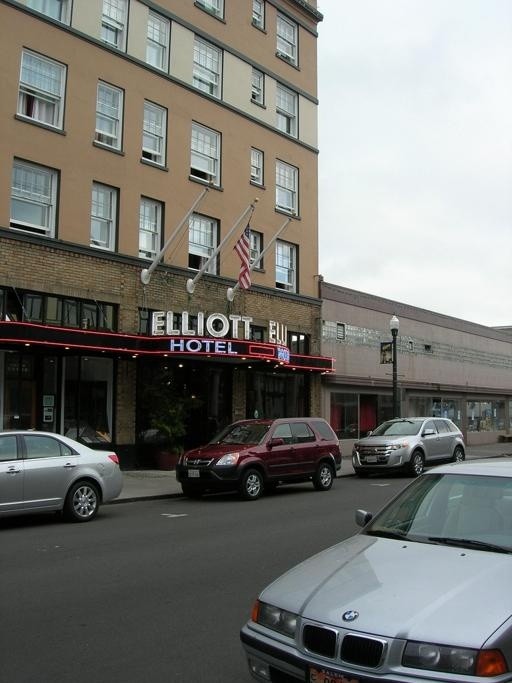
0, 427, 127, 526
240, 451, 512, 682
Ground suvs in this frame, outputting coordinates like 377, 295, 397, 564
172, 414, 344, 499
348, 414, 467, 479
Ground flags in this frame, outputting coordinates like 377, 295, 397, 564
234, 222, 253, 292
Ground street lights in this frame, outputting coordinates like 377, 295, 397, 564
389, 313, 403, 417
